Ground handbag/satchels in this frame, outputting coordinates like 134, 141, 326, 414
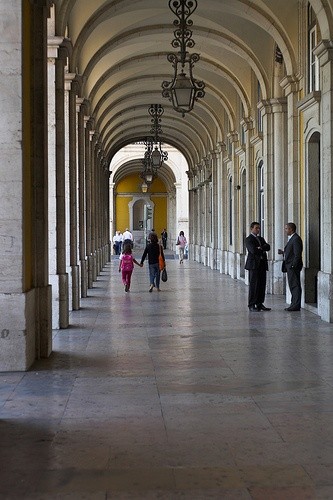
162, 269, 168, 282
176, 237, 179, 245
160, 245, 164, 269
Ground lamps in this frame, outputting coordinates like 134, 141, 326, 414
161, 0, 206, 117
140, 137, 157, 185
145, 103, 169, 170
141, 181, 148, 193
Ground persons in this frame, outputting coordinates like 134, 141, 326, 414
243, 222, 272, 312
161, 228, 167, 250
112, 228, 134, 255
278, 223, 303, 311
118, 244, 142, 292
177, 231, 187, 264
140, 234, 166, 293
149, 228, 158, 240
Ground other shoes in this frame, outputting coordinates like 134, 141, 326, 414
180, 260, 184, 264
149, 285, 154, 292
157, 289, 160, 291
125, 284, 129, 292
285, 307, 301, 311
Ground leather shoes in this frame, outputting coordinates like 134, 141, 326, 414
257, 305, 271, 310
249, 306, 261, 312
